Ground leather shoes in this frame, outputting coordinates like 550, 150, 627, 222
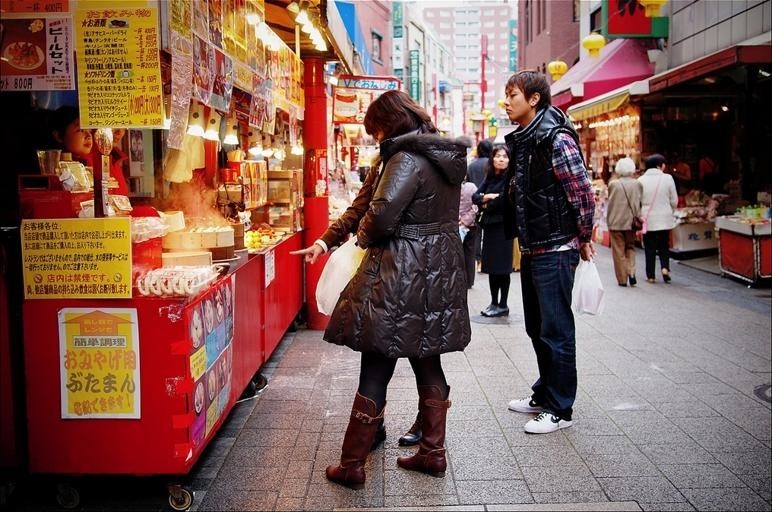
485, 304, 509, 317
481, 303, 496, 315
399, 413, 422, 445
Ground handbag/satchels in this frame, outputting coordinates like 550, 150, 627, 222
632, 215, 643, 230
641, 216, 647, 234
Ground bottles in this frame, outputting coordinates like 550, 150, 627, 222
736, 203, 770, 221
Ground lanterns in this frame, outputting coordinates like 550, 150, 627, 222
639, 0, 668, 19
498, 99, 506, 110
466, 110, 477, 128
482, 109, 492, 124
547, 57, 569, 81
581, 31, 606, 59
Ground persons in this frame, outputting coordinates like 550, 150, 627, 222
44, 101, 92, 163
501, 65, 598, 437
78, 126, 134, 199
288, 138, 520, 453
637, 152, 681, 286
606, 156, 644, 288
324, 87, 471, 491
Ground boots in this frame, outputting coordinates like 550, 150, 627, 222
326, 392, 388, 489
398, 385, 450, 477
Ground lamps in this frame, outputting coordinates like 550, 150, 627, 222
162, 91, 287, 160
297, 11, 328, 53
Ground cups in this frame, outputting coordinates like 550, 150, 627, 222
36, 150, 61, 176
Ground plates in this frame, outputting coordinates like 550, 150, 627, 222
3, 41, 45, 70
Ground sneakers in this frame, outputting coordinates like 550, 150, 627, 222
524, 410, 572, 433
619, 268, 671, 286
507, 397, 541, 413
